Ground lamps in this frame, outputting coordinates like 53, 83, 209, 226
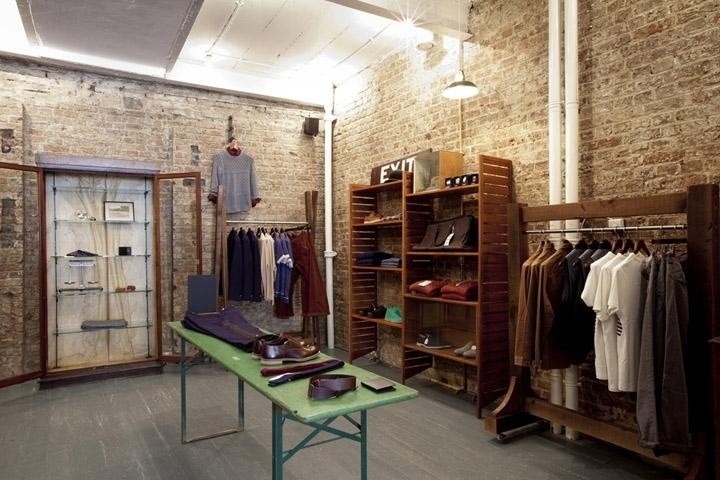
444, 1, 480, 100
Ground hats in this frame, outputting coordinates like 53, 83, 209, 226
384, 169, 402, 183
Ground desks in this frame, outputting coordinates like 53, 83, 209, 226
167, 318, 419, 479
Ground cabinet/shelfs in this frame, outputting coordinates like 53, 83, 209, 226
349, 171, 413, 383
0, 162, 202, 391
406, 153, 512, 418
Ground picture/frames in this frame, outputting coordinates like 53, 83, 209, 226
104, 201, 135, 221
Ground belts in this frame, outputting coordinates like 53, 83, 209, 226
308, 372, 356, 399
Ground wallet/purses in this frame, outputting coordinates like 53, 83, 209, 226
361, 376, 396, 394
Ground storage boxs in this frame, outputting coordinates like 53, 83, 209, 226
412, 150, 466, 193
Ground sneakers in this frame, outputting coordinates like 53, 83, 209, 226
368, 303, 386, 318
454, 340, 475, 355
363, 214, 386, 224
392, 305, 403, 318
359, 303, 378, 315
384, 307, 402, 324
463, 346, 476, 358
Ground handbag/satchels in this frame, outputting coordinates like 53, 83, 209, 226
412, 215, 477, 251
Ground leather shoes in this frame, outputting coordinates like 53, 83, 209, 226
261, 335, 320, 365
252, 332, 312, 359
416, 327, 443, 349
433, 334, 452, 348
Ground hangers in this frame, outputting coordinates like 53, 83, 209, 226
231, 221, 235, 230
538, 230, 545, 250
587, 228, 600, 251
227, 138, 241, 154
248, 221, 252, 232
279, 222, 284, 233
256, 221, 262, 237
544, 230, 552, 246
575, 228, 588, 251
239, 221, 244, 230
613, 227, 623, 254
261, 221, 266, 234
269, 221, 275, 235
265, 221, 268, 233
274, 221, 279, 233
559, 230, 571, 249
634, 226, 651, 259
600, 228, 613, 251
624, 227, 636, 254
